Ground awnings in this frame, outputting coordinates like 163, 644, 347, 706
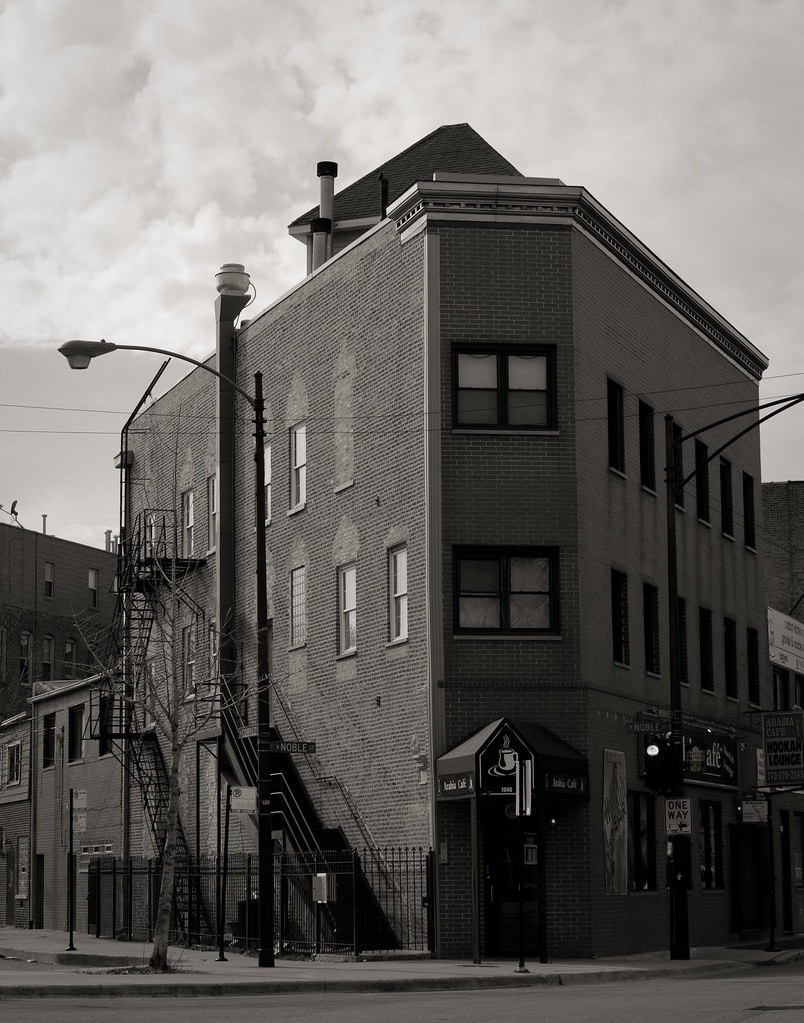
435, 715, 590, 802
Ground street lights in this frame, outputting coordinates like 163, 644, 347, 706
59, 334, 279, 969
